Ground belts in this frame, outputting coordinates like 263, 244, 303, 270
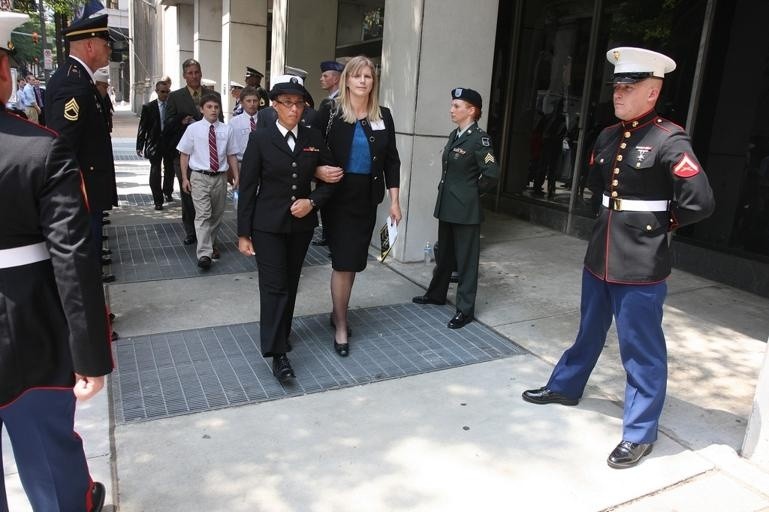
25, 106, 35, 108
193, 170, 228, 176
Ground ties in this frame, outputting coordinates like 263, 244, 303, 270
161, 102, 166, 132
210, 125, 219, 172
193, 91, 201, 117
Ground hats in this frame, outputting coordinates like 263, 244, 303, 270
1, 11, 29, 53
451, 88, 482, 107
63, 14, 117, 43
200, 61, 345, 90
606, 46, 677, 83
95, 72, 109, 84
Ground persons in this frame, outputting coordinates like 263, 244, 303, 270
526, 94, 574, 201
236, 57, 405, 384
0, 1, 128, 512
411, 84, 502, 330
136, 58, 270, 269
522, 44, 716, 469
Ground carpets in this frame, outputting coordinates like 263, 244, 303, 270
117, 193, 234, 207
112, 138, 146, 160
103, 219, 377, 284
111, 302, 528, 426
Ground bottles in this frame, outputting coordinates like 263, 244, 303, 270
424, 242, 433, 265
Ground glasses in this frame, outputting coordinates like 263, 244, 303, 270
278, 101, 305, 108
186, 71, 200, 75
158, 90, 171, 93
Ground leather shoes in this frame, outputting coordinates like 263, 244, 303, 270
104, 211, 119, 342
522, 386, 579, 405
448, 311, 474, 329
412, 296, 447, 304
607, 441, 653, 469
333, 337, 349, 356
155, 195, 220, 269
330, 312, 352, 337
91, 482, 105, 512
273, 354, 296, 382
